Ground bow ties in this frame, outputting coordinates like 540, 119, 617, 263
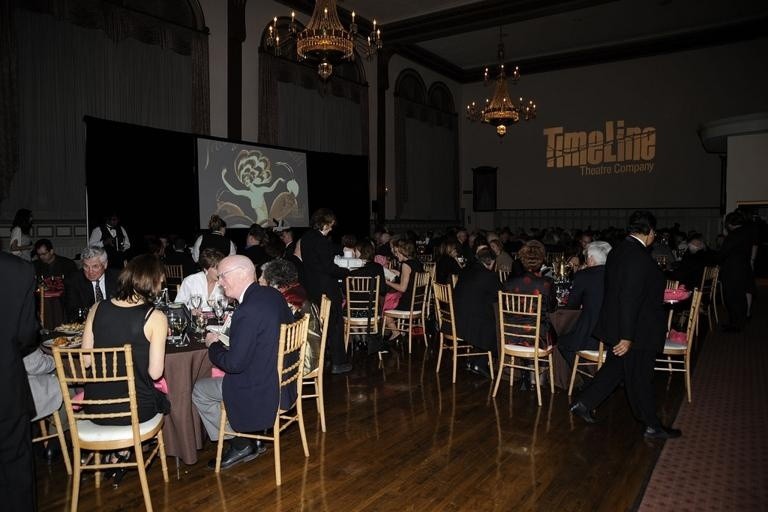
107, 226, 116, 230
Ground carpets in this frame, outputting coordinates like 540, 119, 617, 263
631, 308, 768, 512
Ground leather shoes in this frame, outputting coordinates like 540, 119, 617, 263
208, 441, 259, 469
645, 425, 681, 438
255, 440, 266, 453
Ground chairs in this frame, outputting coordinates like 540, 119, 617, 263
654, 287, 703, 403
422, 262, 436, 320
568, 342, 606, 396
432, 282, 494, 383
492, 290, 555, 406
680, 266, 719, 332
382, 272, 431, 354
299, 414, 329, 512
372, 347, 429, 433
31, 409, 74, 475
51, 344, 169, 512
214, 312, 310, 486
343, 275, 382, 361
165, 264, 184, 292
345, 374, 380, 446
263, 294, 331, 434
492, 386, 551, 496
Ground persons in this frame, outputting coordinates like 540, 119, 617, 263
569, 210, 682, 444
169, 239, 189, 264
670, 233, 687, 261
332, 234, 358, 262
174, 249, 229, 312
387, 240, 427, 344
260, 232, 283, 258
348, 241, 388, 349
379, 233, 389, 250
63, 246, 124, 324
435, 240, 463, 284
192, 215, 236, 262
389, 232, 410, 257
33, 239, 85, 283
493, 238, 515, 275
81, 255, 169, 485
721, 212, 753, 330
437, 239, 458, 295
157, 235, 167, 250
285, 238, 304, 267
373, 229, 383, 241
444, 247, 502, 377
474, 236, 493, 272
571, 234, 593, 266
744, 198, 768, 318
9, 209, 35, 261
455, 231, 467, 248
257, 260, 319, 372
241, 224, 270, 265
23, 348, 77, 459
676, 234, 715, 298
0, 251, 41, 503
192, 256, 300, 471
89, 211, 130, 252
142, 238, 164, 261
503, 240, 558, 394
300, 208, 350, 375
280, 228, 295, 245
558, 241, 612, 388
665, 223, 683, 245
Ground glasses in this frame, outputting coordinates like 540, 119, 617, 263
217, 266, 243, 280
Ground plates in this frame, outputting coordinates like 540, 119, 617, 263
206, 325, 223, 333
44, 337, 82, 348
55, 324, 83, 334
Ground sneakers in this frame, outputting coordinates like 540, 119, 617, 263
332, 363, 352, 374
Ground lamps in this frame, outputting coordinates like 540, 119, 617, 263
266, 1, 383, 79
466, 43, 537, 143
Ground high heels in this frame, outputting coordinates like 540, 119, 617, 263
387, 333, 403, 345
104, 449, 125, 480
113, 465, 128, 488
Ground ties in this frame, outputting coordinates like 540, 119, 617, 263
94, 281, 104, 303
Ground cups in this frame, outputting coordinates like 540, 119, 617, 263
218, 297, 229, 322
205, 294, 216, 318
215, 302, 225, 328
190, 293, 201, 322
176, 317, 188, 346
197, 313, 209, 343
168, 314, 181, 343
